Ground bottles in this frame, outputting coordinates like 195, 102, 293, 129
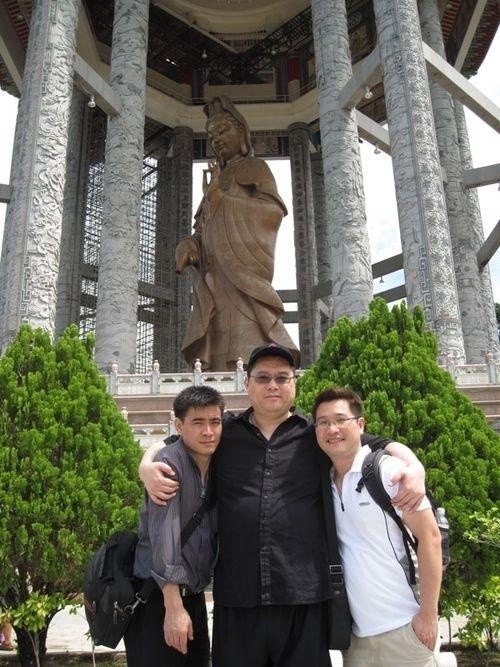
435, 507, 450, 565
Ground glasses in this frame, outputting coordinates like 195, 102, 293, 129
314, 416, 362, 428
250, 375, 294, 383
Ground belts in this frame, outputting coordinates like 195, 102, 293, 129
151, 587, 194, 597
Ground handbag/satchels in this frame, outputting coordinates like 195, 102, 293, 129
325, 562, 352, 650
83, 530, 140, 649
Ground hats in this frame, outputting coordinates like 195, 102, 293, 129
248, 344, 296, 371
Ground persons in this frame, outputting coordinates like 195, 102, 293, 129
0, 622, 14, 651
137, 345, 426, 666
175, 97, 301, 372
124, 385, 225, 667
312, 388, 442, 667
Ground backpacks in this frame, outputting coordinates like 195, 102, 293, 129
356, 449, 451, 572
